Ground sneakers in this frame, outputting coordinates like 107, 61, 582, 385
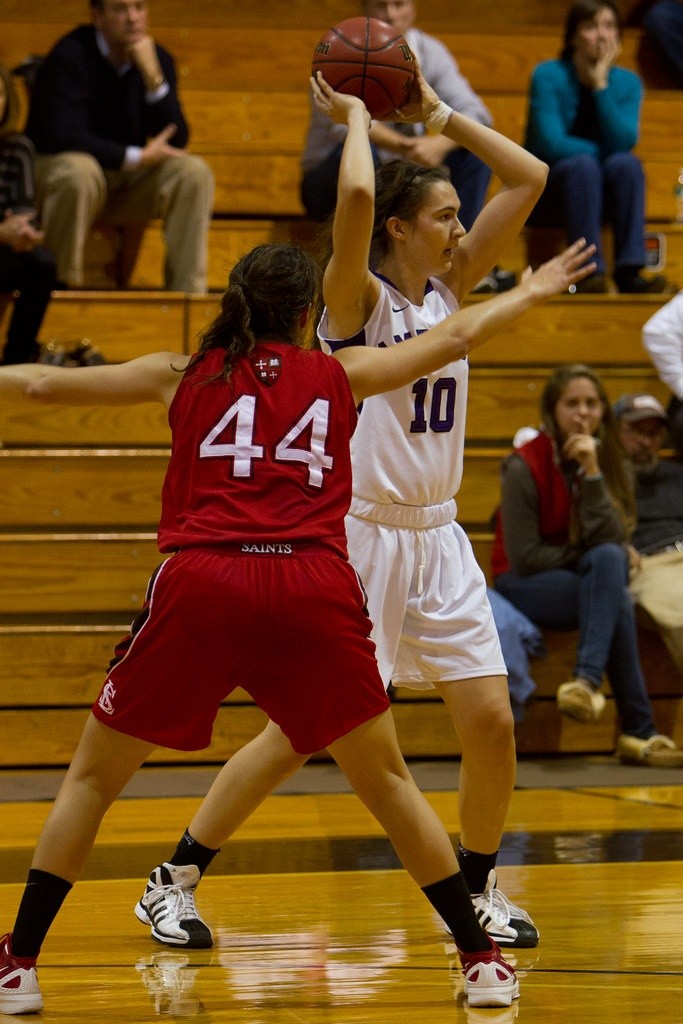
442, 868, 541, 949
133, 861, 215, 949
136, 954, 204, 1018
0, 932, 45, 1015
454, 929, 521, 1007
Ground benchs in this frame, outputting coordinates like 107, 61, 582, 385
0, 0, 683, 770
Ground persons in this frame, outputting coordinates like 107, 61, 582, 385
28, 0, 212, 290
524, 0, 683, 294
610, 394, 683, 674
0, 239, 598, 1014
490, 366, 683, 768
133, 72, 549, 948
642, 289, 683, 459
300, 0, 517, 293
0, 63, 59, 365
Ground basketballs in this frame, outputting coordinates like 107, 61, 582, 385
311, 17, 414, 120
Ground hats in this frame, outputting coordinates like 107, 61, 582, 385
608, 393, 668, 424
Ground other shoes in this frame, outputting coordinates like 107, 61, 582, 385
615, 733, 683, 770
469, 265, 518, 294
555, 681, 607, 723
612, 265, 669, 295
569, 271, 607, 294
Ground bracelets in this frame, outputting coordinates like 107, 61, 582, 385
425, 100, 453, 134
147, 77, 164, 88
